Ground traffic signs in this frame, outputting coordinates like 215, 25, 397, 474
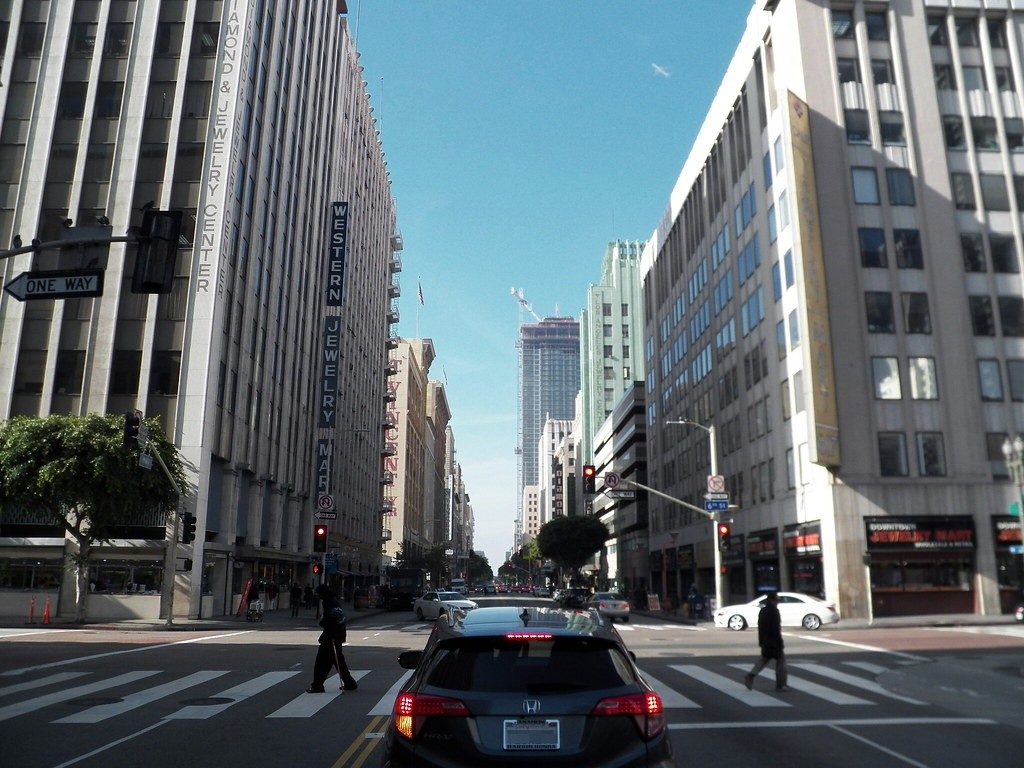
605, 489, 636, 501
313, 510, 337, 520
2, 268, 103, 301
702, 491, 729, 501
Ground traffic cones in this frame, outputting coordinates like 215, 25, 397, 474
40, 603, 50, 625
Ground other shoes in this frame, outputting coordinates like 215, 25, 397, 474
743, 673, 753, 690
340, 685, 357, 690
777, 686, 792, 691
306, 688, 325, 693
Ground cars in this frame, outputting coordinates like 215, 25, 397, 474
383, 606, 671, 768
414, 592, 478, 622
465, 582, 550, 598
713, 592, 840, 631
552, 588, 630, 623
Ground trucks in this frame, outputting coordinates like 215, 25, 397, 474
451, 579, 466, 595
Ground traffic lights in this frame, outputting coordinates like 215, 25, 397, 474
722, 562, 727, 574
717, 522, 730, 552
311, 524, 328, 556
583, 465, 595, 494
313, 563, 323, 575
508, 562, 512, 570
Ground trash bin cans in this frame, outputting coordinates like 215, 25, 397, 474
688, 595, 705, 618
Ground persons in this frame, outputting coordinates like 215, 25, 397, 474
304, 584, 313, 609
381, 584, 390, 609
266, 581, 278, 610
246, 582, 259, 609
745, 593, 791, 692
306, 585, 358, 693
290, 583, 303, 618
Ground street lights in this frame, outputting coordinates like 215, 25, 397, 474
667, 417, 723, 608
1001, 434, 1024, 544
